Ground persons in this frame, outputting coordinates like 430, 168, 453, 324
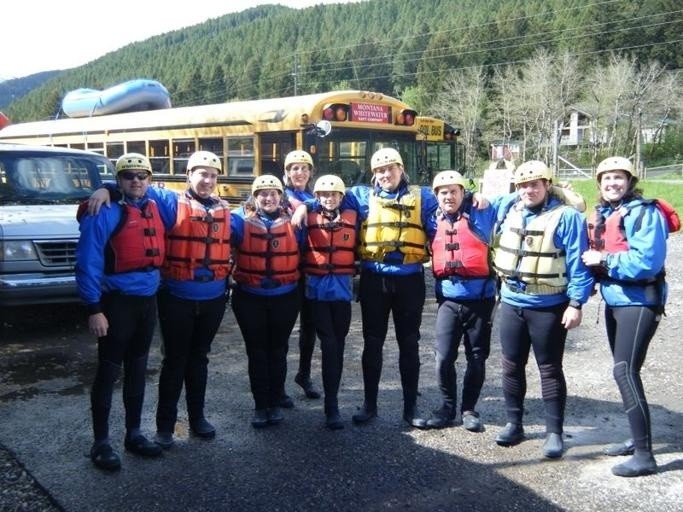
87, 150, 234, 448
294, 174, 360, 430
580, 155, 669, 477
424, 170, 495, 430
74, 153, 165, 471
227, 175, 301, 428
485, 162, 596, 458
281, 150, 321, 409
290, 149, 490, 429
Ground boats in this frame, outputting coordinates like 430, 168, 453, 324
59, 80, 170, 118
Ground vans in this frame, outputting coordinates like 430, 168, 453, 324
0, 143, 123, 308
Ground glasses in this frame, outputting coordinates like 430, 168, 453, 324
120, 172, 149, 179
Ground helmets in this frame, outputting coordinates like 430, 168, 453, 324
285, 150, 313, 171
515, 160, 550, 188
116, 153, 152, 175
313, 175, 346, 195
251, 175, 284, 197
371, 148, 403, 173
596, 157, 639, 183
433, 170, 465, 195
186, 151, 222, 175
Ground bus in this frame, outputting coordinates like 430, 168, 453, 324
338, 115, 468, 187
0, 89, 421, 286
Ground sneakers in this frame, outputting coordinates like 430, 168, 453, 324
297, 375, 321, 400
91, 442, 120, 470
189, 418, 216, 438
604, 439, 657, 476
352, 403, 377, 422
497, 421, 524, 443
251, 395, 294, 427
124, 435, 159, 457
542, 434, 563, 458
326, 409, 346, 431
154, 428, 174, 449
403, 406, 481, 431
